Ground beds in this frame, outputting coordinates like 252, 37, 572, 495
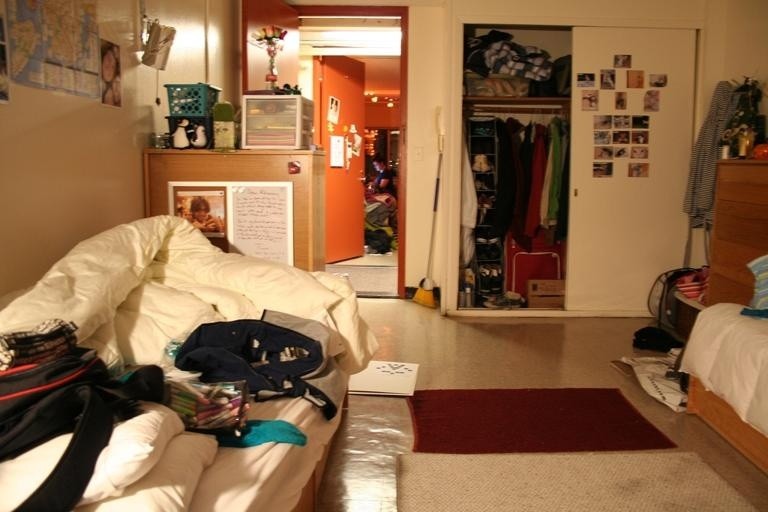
1, 209, 382, 512
677, 299, 768, 473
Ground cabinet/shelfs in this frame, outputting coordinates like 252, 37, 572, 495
704, 156, 768, 310
467, 115, 505, 307
140, 147, 328, 273
446, 18, 698, 318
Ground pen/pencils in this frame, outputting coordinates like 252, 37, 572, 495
166, 379, 250, 430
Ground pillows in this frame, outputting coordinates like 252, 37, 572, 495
70, 430, 219, 512
1, 395, 186, 512
737, 253, 768, 317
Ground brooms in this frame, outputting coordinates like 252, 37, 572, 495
411, 154, 445, 310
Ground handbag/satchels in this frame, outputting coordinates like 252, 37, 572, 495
0, 316, 140, 512
174, 319, 323, 401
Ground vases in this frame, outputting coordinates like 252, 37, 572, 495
264, 45, 280, 93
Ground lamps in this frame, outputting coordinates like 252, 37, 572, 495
141, 20, 177, 74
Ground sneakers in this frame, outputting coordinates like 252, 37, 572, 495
471, 154, 502, 292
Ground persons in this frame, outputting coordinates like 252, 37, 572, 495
577, 55, 649, 177
369, 155, 396, 201
176, 196, 221, 233
329, 99, 337, 122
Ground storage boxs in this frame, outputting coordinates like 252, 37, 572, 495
527, 278, 564, 307
460, 68, 531, 98
239, 93, 315, 151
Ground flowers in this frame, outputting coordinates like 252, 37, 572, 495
251, 22, 289, 90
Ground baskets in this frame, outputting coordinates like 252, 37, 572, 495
163, 83, 223, 149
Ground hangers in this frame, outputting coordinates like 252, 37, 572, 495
475, 105, 568, 126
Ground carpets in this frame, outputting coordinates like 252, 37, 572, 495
404, 384, 681, 453
394, 450, 759, 511
322, 263, 399, 299
